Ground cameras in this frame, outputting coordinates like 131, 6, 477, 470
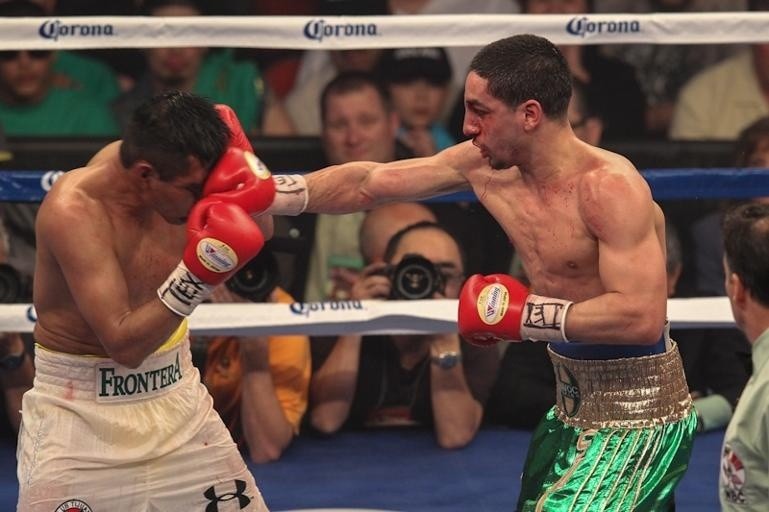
224, 245, 283, 303
375, 253, 441, 300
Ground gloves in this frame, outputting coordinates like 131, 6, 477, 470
458, 274, 574, 347
204, 147, 309, 218
213, 104, 255, 154
157, 199, 265, 318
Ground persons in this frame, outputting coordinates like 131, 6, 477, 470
0, 0, 767, 462
14, 92, 277, 511
715, 198, 769, 511
208, 34, 697, 511
